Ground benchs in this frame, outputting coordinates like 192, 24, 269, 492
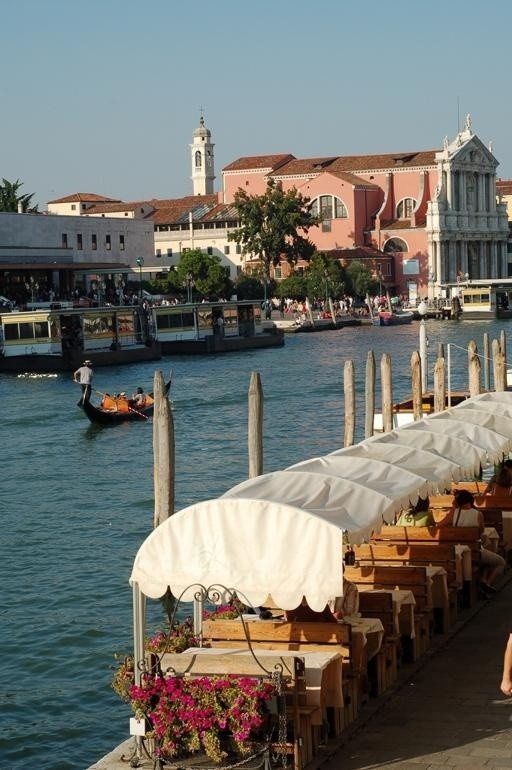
142, 482, 512, 770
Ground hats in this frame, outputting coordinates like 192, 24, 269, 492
84, 360, 93, 365
119, 392, 127, 397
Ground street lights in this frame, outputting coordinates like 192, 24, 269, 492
416, 300, 430, 395
136, 256, 144, 296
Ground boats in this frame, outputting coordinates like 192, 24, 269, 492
372, 310, 414, 327
1, 304, 151, 372
460, 286, 512, 320
76, 369, 175, 425
151, 297, 286, 355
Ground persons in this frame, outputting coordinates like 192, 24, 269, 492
213, 307, 224, 340
499, 628, 512, 700
59, 319, 83, 359
132, 387, 146, 408
218, 296, 224, 302
102, 393, 115, 410
395, 496, 437, 526
491, 468, 512, 497
286, 596, 338, 623
201, 297, 206, 304
262, 292, 404, 326
1, 273, 188, 311
74, 360, 94, 406
440, 489, 468, 523
116, 392, 130, 413
438, 492, 507, 601
223, 297, 228, 302
475, 459, 512, 505
327, 562, 360, 620
